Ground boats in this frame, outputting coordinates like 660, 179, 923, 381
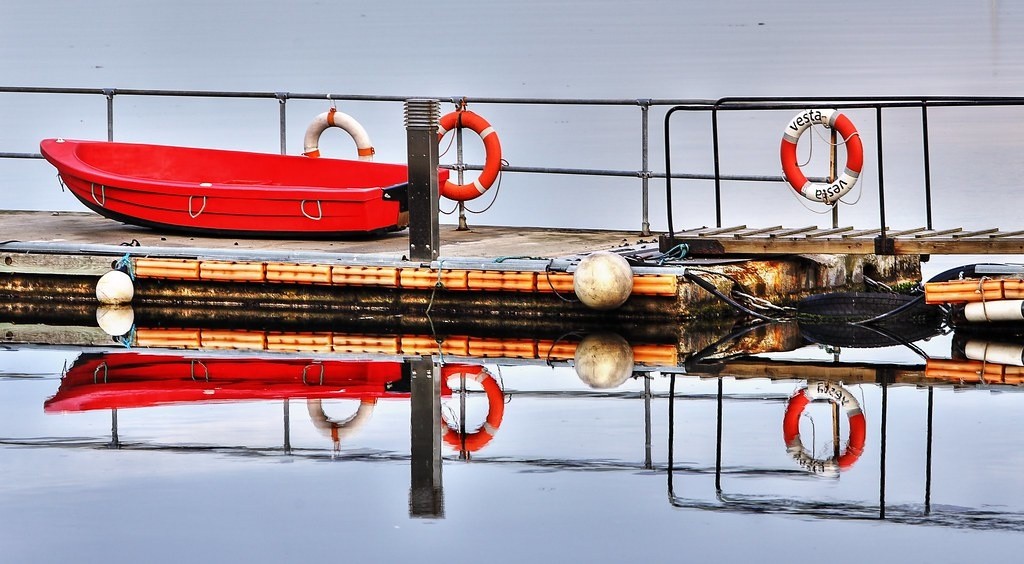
39, 138, 450, 239
43, 351, 452, 414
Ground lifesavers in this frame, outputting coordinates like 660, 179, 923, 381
780, 109, 864, 203
303, 108, 375, 162
438, 110, 502, 201
440, 363, 504, 452
307, 398, 376, 440
782, 381, 866, 475
